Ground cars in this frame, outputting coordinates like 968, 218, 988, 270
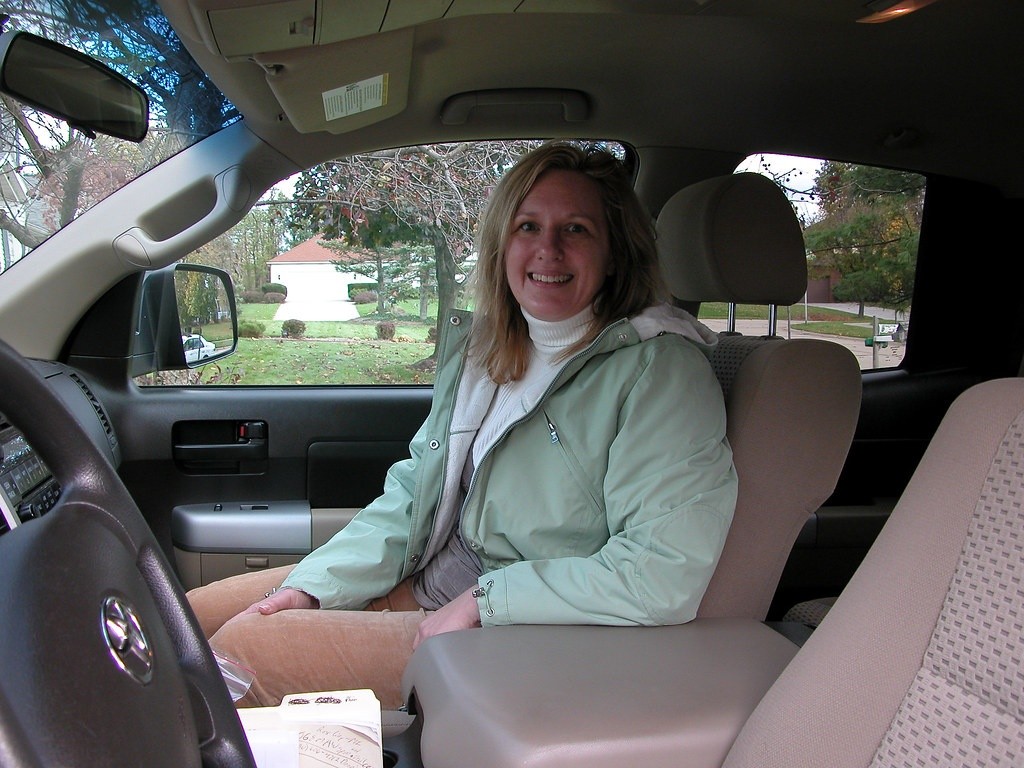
182, 333, 216, 361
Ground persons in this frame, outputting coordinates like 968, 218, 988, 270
184, 140, 739, 709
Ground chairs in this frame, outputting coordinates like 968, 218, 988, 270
728, 375, 1024, 768
654, 171, 866, 628
